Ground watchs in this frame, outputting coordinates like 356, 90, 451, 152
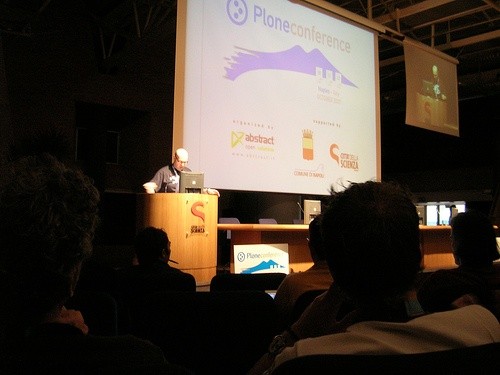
268, 334, 291, 358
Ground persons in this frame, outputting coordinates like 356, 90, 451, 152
117, 227, 195, 375
417, 210, 500, 325
142, 148, 220, 197
247, 181, 500, 375
0, 152, 170, 375
432, 65, 447, 101
274, 214, 334, 305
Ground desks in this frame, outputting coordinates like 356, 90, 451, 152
218, 224, 460, 273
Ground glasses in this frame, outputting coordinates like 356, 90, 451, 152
174, 157, 188, 164
307, 235, 312, 243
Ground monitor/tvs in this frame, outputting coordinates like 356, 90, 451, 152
179, 171, 204, 194
304, 200, 321, 225
423, 80, 434, 98
416, 201, 466, 227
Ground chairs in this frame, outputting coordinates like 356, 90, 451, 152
1, 264, 500, 374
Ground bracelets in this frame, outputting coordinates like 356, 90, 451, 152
286, 325, 299, 341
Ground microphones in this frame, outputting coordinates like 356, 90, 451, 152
297, 202, 304, 213
